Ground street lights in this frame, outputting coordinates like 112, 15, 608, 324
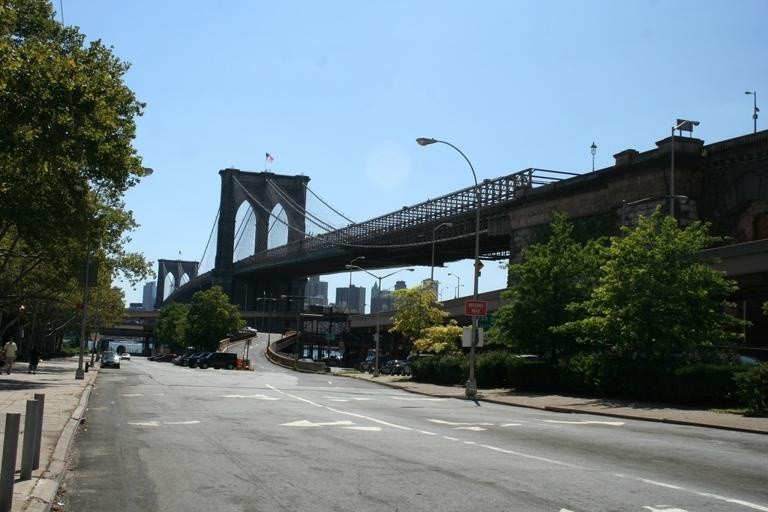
281, 295, 324, 360
590, 141, 597, 173
744, 91, 757, 132
416, 137, 484, 396
347, 256, 366, 330
447, 272, 464, 298
622, 194, 688, 239
431, 222, 454, 280
256, 291, 276, 328
671, 120, 700, 217
346, 264, 415, 377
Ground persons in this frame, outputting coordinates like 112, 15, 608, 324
1, 336, 18, 374
27, 344, 41, 375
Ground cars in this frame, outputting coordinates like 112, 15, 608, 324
100, 351, 120, 368
148, 352, 237, 369
239, 327, 258, 333
360, 356, 410, 376
121, 353, 130, 360
